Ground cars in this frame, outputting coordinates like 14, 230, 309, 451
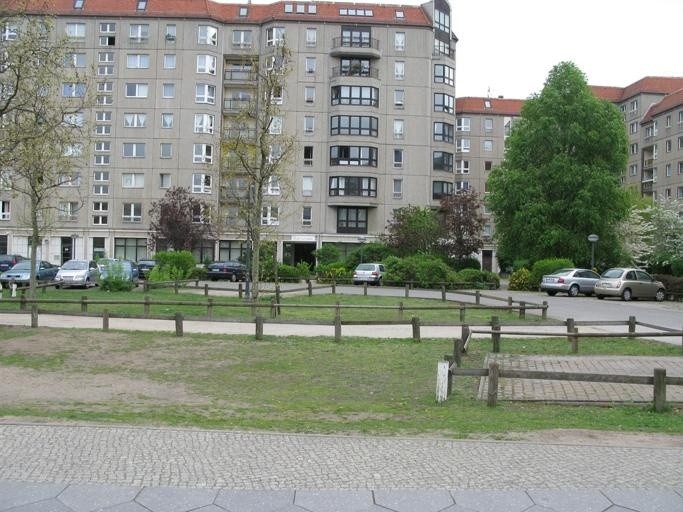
55, 259, 99, 289
539, 268, 600, 297
207, 259, 246, 282
98, 260, 138, 284
97, 258, 117, 273
352, 263, 385, 286
593, 267, 666, 302
0, 259, 59, 289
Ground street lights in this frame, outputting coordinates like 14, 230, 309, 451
357, 236, 365, 263
70, 232, 78, 259
587, 233, 598, 269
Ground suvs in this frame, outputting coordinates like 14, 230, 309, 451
0, 255, 25, 272
136, 260, 156, 279
